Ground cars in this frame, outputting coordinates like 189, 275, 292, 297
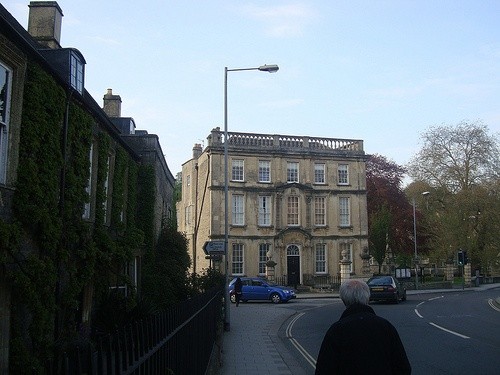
366, 274, 407, 304
229, 277, 297, 303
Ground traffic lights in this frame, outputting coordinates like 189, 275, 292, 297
457, 250, 464, 266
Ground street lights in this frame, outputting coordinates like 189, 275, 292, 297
413, 191, 431, 289
224, 64, 280, 332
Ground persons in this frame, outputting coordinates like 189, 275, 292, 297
235, 278, 243, 307
314, 279, 412, 375
